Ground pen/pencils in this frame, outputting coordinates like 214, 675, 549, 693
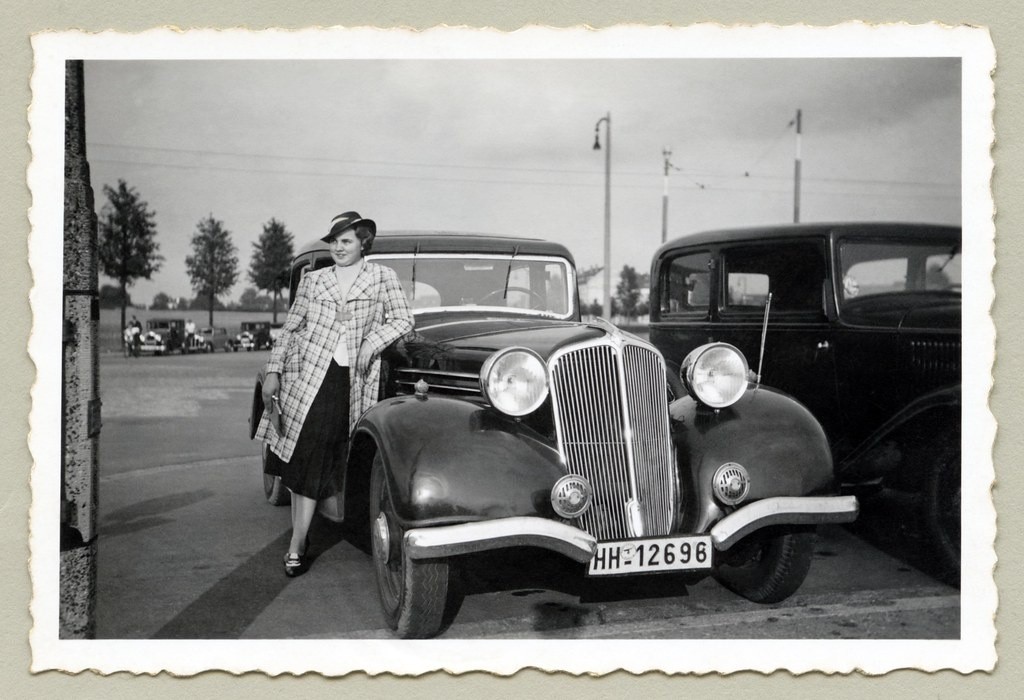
272, 395, 278, 400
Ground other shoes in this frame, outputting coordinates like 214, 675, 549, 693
285, 543, 306, 576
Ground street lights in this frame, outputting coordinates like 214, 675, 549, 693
592, 111, 613, 323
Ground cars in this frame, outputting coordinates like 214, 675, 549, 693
650, 221, 961, 593
224, 321, 273, 352
179, 327, 229, 355
248, 230, 862, 641
138, 316, 185, 357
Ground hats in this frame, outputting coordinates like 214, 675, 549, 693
319, 213, 376, 243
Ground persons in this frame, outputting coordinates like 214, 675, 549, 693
127, 314, 142, 343
185, 319, 196, 342
123, 323, 139, 358
255, 211, 416, 575
